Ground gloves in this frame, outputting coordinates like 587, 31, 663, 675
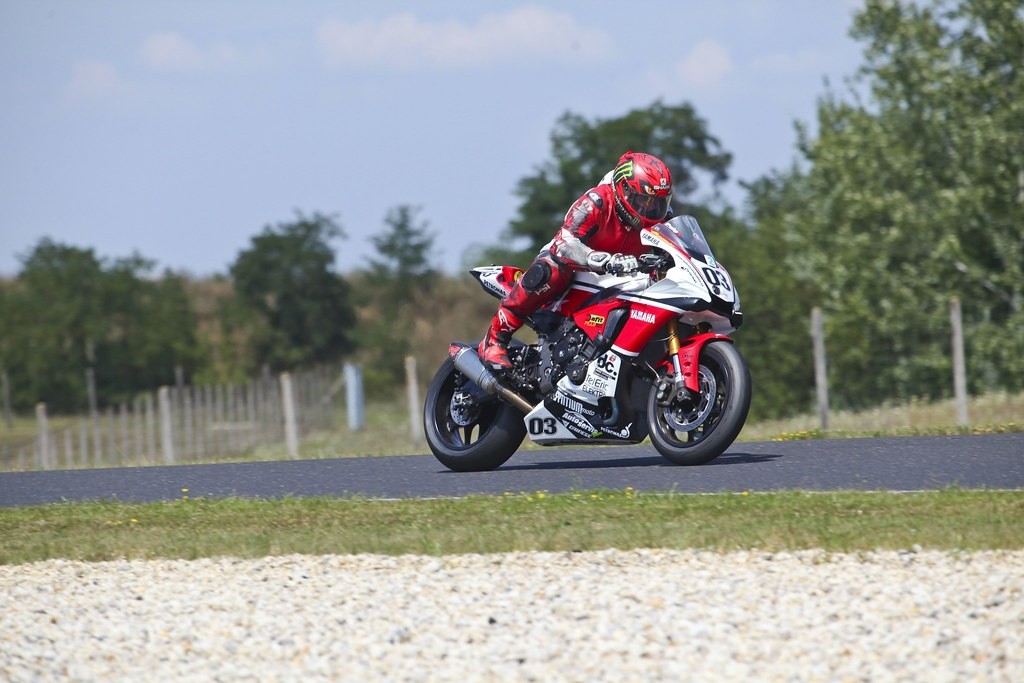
607, 252, 639, 277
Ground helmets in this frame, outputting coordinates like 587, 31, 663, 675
612, 152, 673, 233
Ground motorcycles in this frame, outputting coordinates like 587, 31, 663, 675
422, 216, 752, 474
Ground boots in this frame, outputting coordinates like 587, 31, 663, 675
477, 303, 524, 371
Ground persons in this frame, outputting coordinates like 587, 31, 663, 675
477, 151, 681, 370
